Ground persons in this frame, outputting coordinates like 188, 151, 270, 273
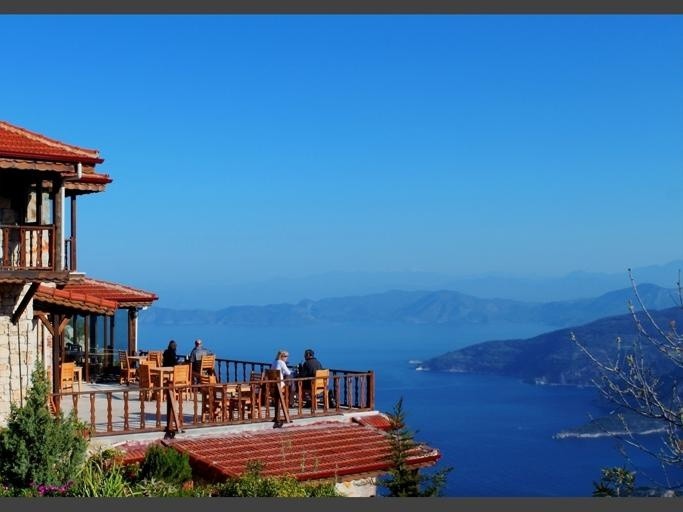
181, 338, 210, 373
162, 340, 180, 366
295, 348, 324, 408
271, 350, 292, 408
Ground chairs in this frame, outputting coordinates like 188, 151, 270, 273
117, 347, 329, 420
59, 361, 75, 401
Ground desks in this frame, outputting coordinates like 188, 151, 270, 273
59, 365, 84, 398
